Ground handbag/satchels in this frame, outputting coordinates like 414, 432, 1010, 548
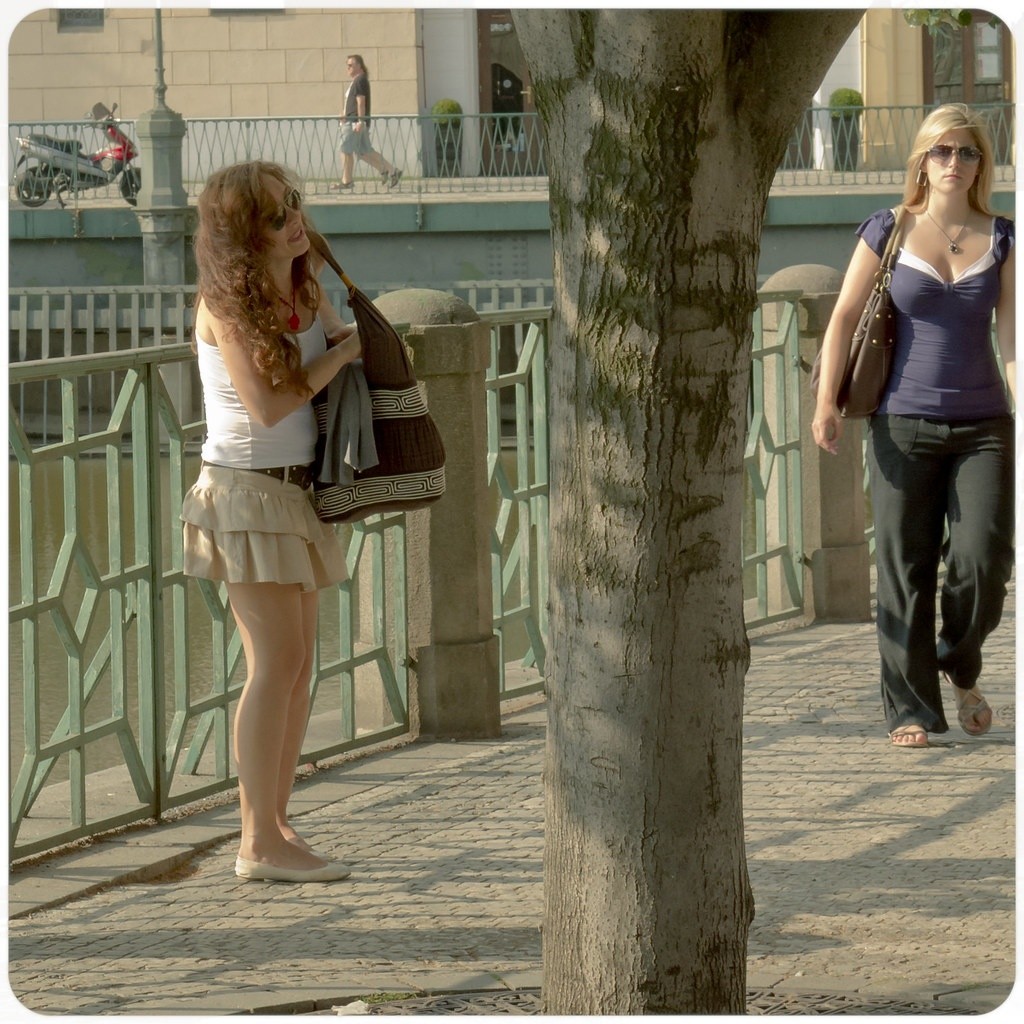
298, 231, 446, 522
809, 204, 907, 415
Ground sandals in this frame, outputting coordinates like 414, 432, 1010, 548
943, 671, 992, 736
888, 724, 929, 747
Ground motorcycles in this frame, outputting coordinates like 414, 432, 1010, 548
14, 102, 141, 208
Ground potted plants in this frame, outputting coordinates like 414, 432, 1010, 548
432, 100, 462, 177
827, 89, 864, 172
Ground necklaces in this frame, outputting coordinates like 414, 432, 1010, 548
280, 292, 300, 330
926, 209, 972, 253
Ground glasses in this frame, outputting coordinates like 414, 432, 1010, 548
255, 185, 303, 236
927, 144, 984, 167
348, 63, 356, 67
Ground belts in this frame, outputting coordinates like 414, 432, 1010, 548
202, 458, 314, 491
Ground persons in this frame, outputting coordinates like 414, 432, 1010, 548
808, 101, 1016, 747
180, 163, 364, 881
329, 55, 401, 189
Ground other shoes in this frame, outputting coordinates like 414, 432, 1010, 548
381, 168, 390, 186
235, 847, 354, 883
390, 165, 403, 187
333, 179, 354, 191
